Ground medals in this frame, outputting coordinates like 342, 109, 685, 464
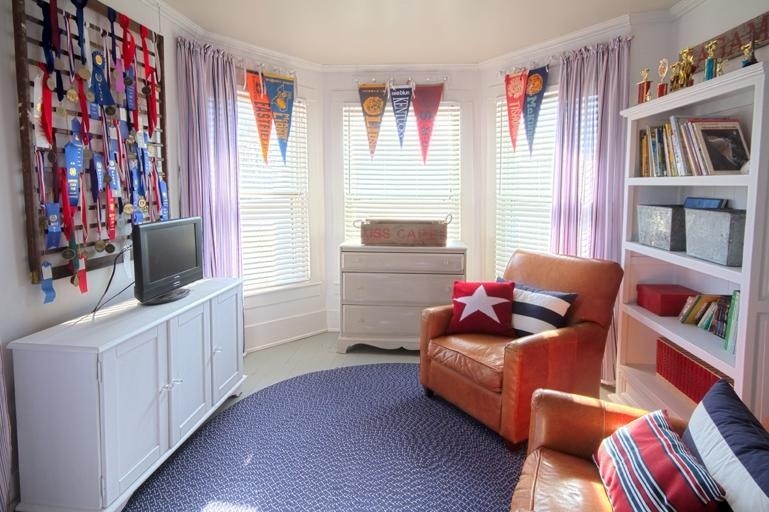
34, 49, 166, 289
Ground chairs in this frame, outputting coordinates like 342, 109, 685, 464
419, 246, 625, 454
508, 386, 768, 512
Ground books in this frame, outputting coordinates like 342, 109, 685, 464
677, 284, 745, 356
635, 113, 748, 176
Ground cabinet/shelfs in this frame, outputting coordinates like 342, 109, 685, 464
337, 238, 467, 356
212, 281, 243, 410
6, 302, 213, 510
609, 62, 767, 433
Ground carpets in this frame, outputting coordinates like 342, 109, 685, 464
117, 361, 526, 511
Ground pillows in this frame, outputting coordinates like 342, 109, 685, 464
494, 274, 579, 339
678, 377, 769, 512
591, 408, 728, 512
445, 278, 518, 340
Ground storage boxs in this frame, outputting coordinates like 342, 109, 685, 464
635, 202, 685, 253
682, 207, 747, 267
636, 283, 705, 316
352, 214, 454, 247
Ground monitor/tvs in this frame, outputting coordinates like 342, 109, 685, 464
132, 216, 203, 305
